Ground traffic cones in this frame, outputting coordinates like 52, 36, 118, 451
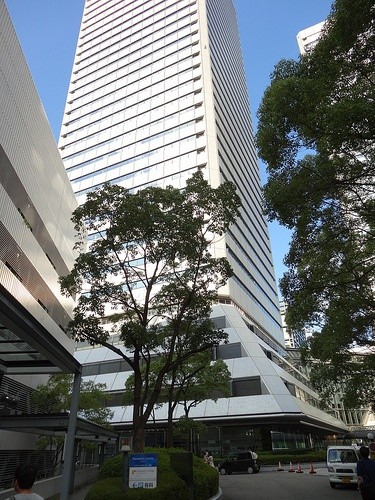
296, 463, 303, 473
309, 463, 317, 473
288, 462, 295, 472
277, 462, 284, 471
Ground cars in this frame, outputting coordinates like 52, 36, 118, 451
218, 450, 261, 475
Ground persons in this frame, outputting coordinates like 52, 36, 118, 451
203, 451, 215, 469
3, 463, 45, 500
356, 442, 375, 500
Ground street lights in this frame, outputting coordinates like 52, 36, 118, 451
367, 433, 374, 450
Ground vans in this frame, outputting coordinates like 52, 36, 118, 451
326, 445, 362, 488
